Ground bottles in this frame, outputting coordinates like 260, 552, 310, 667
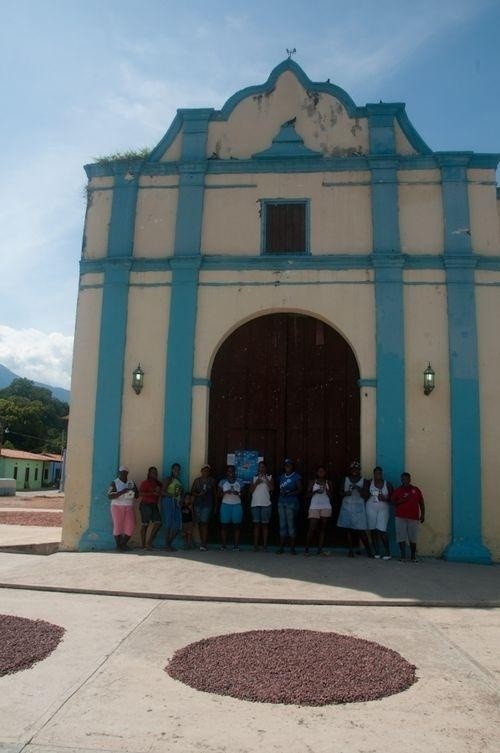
379, 489, 383, 500
348, 484, 354, 493
261, 474, 266, 484
230, 487, 236, 496
319, 481, 324, 493
203, 482, 208, 492
128, 483, 134, 494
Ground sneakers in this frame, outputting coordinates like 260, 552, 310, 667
166, 544, 241, 552
251, 546, 326, 557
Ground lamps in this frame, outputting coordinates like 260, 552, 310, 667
423, 361, 435, 395
132, 362, 143, 393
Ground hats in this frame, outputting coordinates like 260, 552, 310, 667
119, 465, 129, 473
282, 458, 294, 465
349, 459, 361, 469
200, 463, 212, 472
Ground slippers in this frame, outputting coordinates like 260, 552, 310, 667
348, 550, 418, 563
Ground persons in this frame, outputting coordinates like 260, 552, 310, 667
181, 493, 196, 549
160, 463, 184, 549
275, 458, 304, 555
107, 464, 139, 552
191, 464, 218, 551
336, 459, 374, 559
138, 467, 162, 548
218, 465, 245, 551
248, 461, 275, 551
365, 467, 394, 561
391, 472, 425, 563
304, 465, 333, 555
